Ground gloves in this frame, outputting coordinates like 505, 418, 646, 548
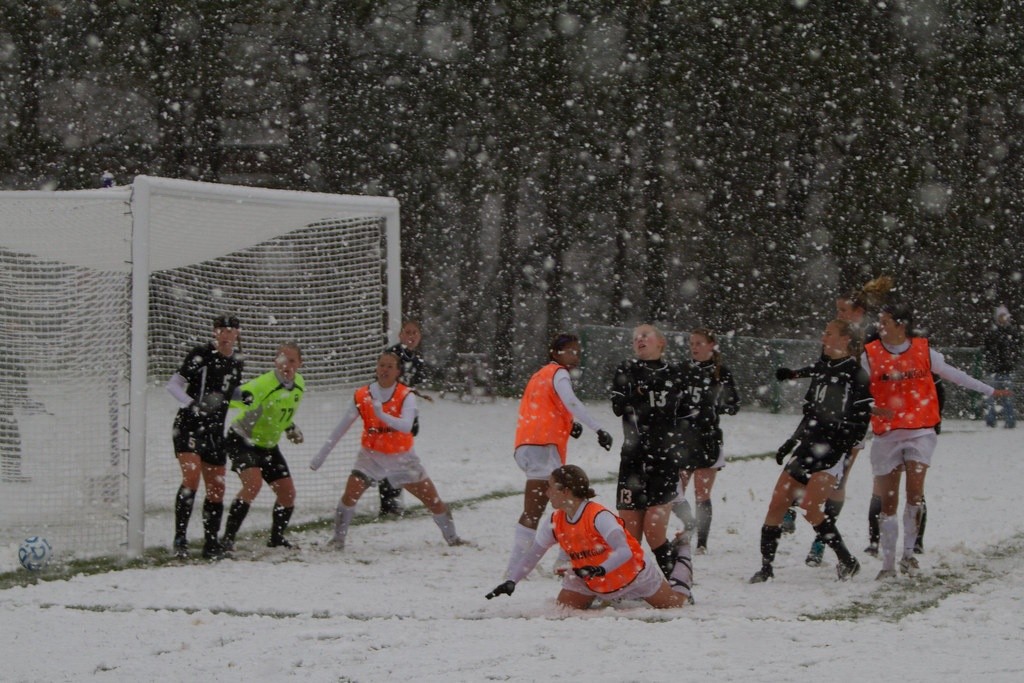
597, 429, 612, 450
776, 367, 796, 381
572, 565, 606, 580
189, 400, 209, 417
485, 580, 515, 599
570, 422, 583, 439
242, 390, 254, 405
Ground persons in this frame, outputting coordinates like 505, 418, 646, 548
219, 342, 305, 550
485, 464, 693, 610
860, 301, 1012, 581
671, 327, 740, 554
165, 315, 254, 557
309, 350, 471, 552
378, 321, 428, 523
504, 334, 613, 581
775, 292, 872, 568
0, 324, 33, 482
986, 307, 1015, 429
610, 324, 682, 581
746, 319, 874, 585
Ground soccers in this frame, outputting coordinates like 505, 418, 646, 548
18, 536, 54, 571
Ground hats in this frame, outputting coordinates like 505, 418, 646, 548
549, 327, 579, 359
213, 315, 240, 328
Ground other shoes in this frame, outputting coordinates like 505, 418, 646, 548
748, 565, 774, 583
173, 539, 190, 559
266, 534, 293, 549
448, 537, 471, 546
805, 538, 825, 566
901, 556, 923, 581
834, 556, 861, 582
203, 541, 226, 560
669, 526, 699, 546
378, 500, 416, 519
777, 531, 795, 554
326, 535, 346, 551
